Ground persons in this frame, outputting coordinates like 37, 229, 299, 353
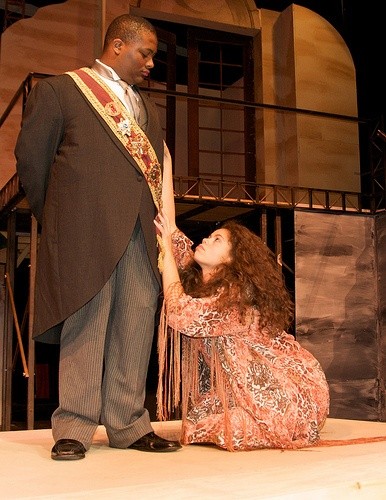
13, 13, 183, 461
153, 138, 331, 452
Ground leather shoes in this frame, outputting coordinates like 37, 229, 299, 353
50, 439, 85, 461
128, 432, 184, 453
